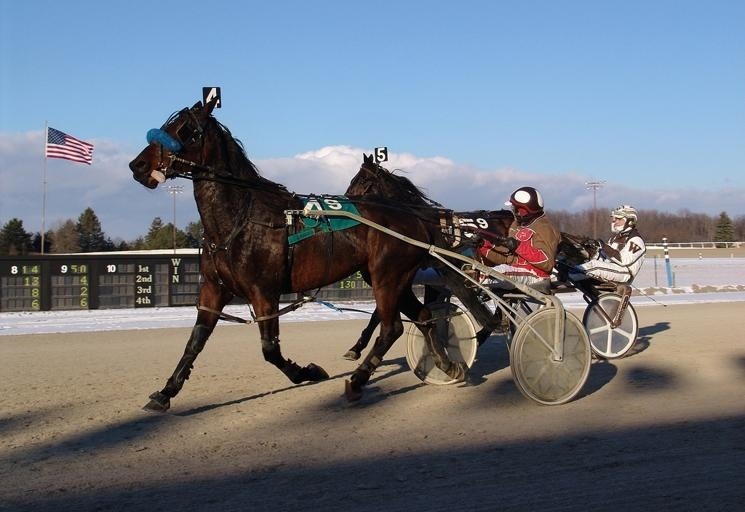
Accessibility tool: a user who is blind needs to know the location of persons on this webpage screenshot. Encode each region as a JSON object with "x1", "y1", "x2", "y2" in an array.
[
  {"x1": 477, "y1": 188, "x2": 562, "y2": 292},
  {"x1": 559, "y1": 207, "x2": 645, "y2": 285}
]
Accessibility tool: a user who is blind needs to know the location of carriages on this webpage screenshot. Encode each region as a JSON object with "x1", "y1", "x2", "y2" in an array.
[{"x1": 129, "y1": 94, "x2": 638, "y2": 414}]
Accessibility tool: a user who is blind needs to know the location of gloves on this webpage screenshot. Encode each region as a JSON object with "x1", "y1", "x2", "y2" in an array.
[
  {"x1": 583, "y1": 239, "x2": 603, "y2": 251},
  {"x1": 470, "y1": 236, "x2": 483, "y2": 248},
  {"x1": 501, "y1": 237, "x2": 520, "y2": 251}
]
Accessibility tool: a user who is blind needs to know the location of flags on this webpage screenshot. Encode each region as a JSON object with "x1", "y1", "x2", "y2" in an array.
[{"x1": 45, "y1": 126, "x2": 95, "y2": 166}]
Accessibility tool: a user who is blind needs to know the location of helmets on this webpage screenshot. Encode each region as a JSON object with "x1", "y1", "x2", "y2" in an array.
[
  {"x1": 609, "y1": 205, "x2": 639, "y2": 235},
  {"x1": 504, "y1": 187, "x2": 544, "y2": 226}
]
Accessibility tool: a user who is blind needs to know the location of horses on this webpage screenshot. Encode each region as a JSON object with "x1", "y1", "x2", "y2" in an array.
[
  {"x1": 340, "y1": 152, "x2": 518, "y2": 361},
  {"x1": 129, "y1": 96, "x2": 468, "y2": 413}
]
